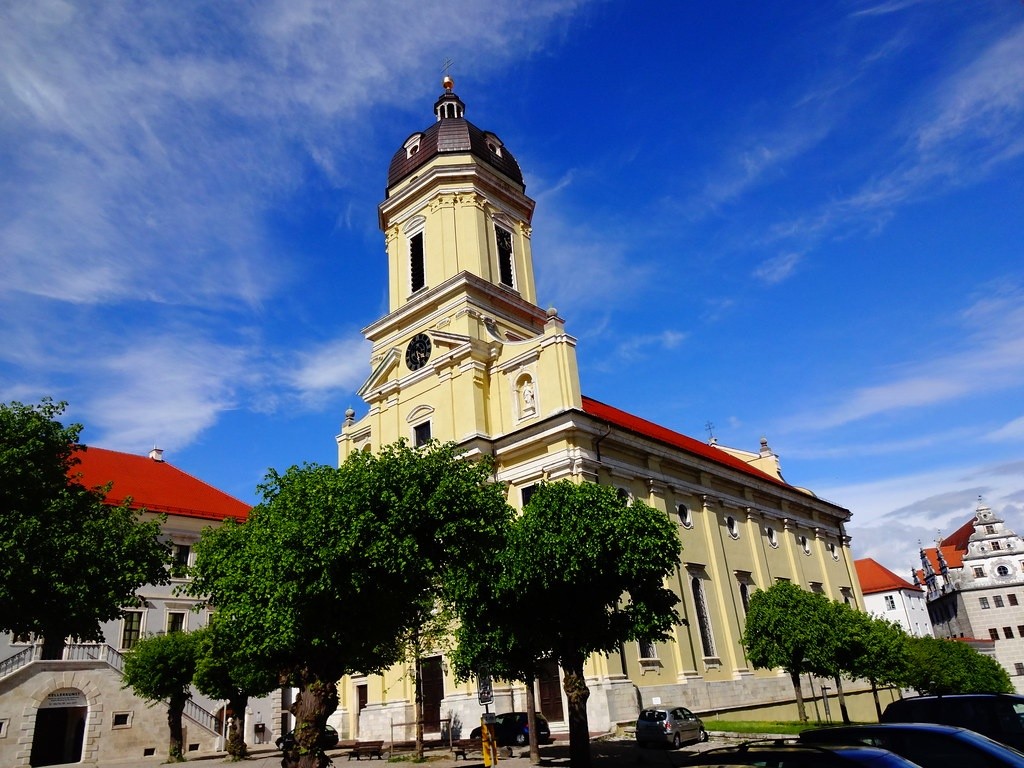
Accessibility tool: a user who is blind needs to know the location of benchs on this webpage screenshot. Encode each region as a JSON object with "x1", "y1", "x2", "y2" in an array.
[
  {"x1": 451, "y1": 737, "x2": 482, "y2": 760},
  {"x1": 348, "y1": 740, "x2": 384, "y2": 761}
]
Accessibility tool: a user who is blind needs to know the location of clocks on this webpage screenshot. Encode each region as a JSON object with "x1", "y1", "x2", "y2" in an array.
[{"x1": 405, "y1": 334, "x2": 432, "y2": 371}]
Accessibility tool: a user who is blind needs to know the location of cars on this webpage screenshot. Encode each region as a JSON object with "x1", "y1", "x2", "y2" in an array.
[
  {"x1": 636, "y1": 705, "x2": 706, "y2": 750},
  {"x1": 672, "y1": 722, "x2": 1024, "y2": 768},
  {"x1": 879, "y1": 692, "x2": 1024, "y2": 754},
  {"x1": 470, "y1": 711, "x2": 551, "y2": 746},
  {"x1": 275, "y1": 724, "x2": 338, "y2": 751}
]
